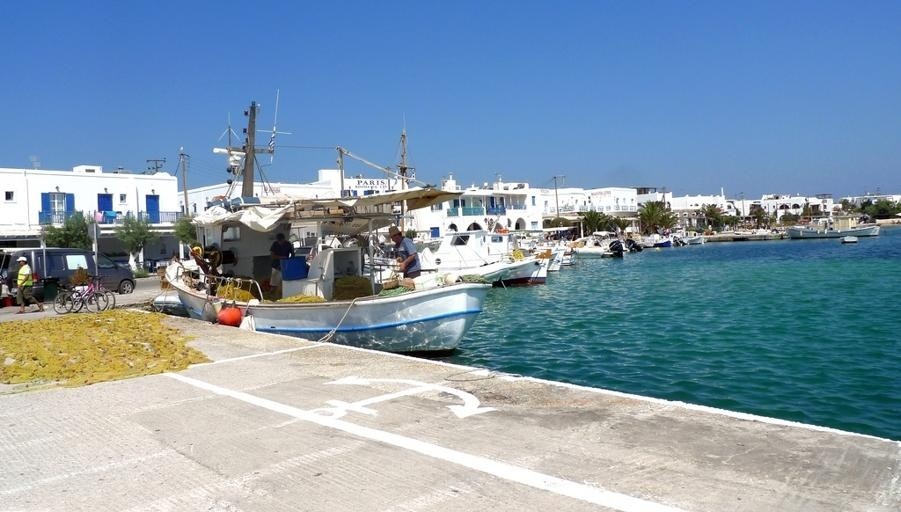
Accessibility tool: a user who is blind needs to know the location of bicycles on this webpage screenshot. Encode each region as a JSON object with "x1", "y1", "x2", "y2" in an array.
[{"x1": 49, "y1": 272, "x2": 120, "y2": 314}]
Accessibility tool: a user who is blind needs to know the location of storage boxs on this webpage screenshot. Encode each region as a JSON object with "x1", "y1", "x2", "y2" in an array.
[{"x1": 278, "y1": 255, "x2": 307, "y2": 281}]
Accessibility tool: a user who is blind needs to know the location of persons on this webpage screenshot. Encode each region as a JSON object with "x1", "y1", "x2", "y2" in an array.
[
  {"x1": 16, "y1": 256, "x2": 43, "y2": 313},
  {"x1": 386, "y1": 226, "x2": 421, "y2": 279},
  {"x1": 269, "y1": 233, "x2": 295, "y2": 298}
]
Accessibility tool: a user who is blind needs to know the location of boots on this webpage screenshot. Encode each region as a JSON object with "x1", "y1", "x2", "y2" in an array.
[
  {"x1": 19, "y1": 305, "x2": 25, "y2": 313},
  {"x1": 38, "y1": 303, "x2": 44, "y2": 312}
]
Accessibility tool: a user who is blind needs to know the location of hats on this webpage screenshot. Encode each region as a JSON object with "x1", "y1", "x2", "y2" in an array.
[
  {"x1": 16, "y1": 255, "x2": 28, "y2": 262},
  {"x1": 387, "y1": 225, "x2": 403, "y2": 239}
]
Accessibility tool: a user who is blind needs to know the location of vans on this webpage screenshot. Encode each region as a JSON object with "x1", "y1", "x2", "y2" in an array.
[{"x1": 0, "y1": 244, "x2": 139, "y2": 305}]
